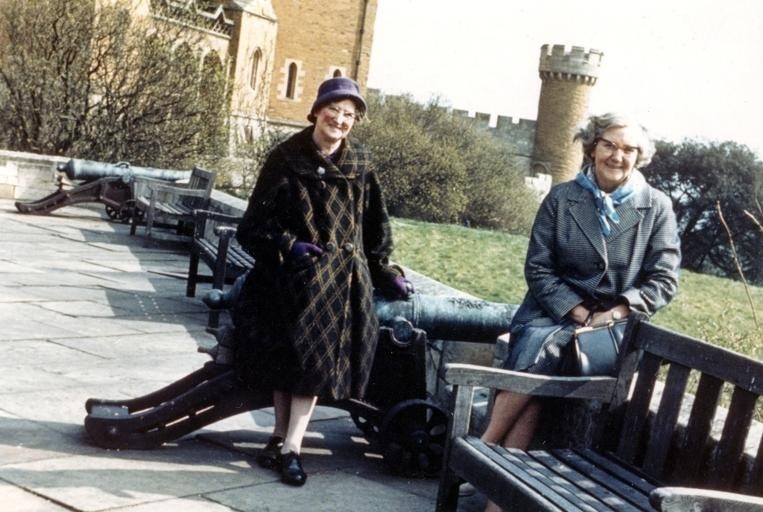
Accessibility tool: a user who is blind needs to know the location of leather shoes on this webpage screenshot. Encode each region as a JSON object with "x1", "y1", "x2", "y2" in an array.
[{"x1": 256, "y1": 436, "x2": 306, "y2": 487}]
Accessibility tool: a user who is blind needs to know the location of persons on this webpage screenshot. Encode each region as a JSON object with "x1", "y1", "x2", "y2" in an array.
[
  {"x1": 236, "y1": 76, "x2": 415, "y2": 486},
  {"x1": 458, "y1": 112, "x2": 683, "y2": 512}
]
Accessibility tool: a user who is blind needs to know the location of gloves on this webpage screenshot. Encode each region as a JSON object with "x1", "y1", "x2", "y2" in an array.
[
  {"x1": 385, "y1": 276, "x2": 415, "y2": 301},
  {"x1": 293, "y1": 242, "x2": 323, "y2": 260}
]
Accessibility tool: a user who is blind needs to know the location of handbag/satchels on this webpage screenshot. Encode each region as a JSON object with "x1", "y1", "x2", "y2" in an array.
[{"x1": 574, "y1": 318, "x2": 634, "y2": 377}]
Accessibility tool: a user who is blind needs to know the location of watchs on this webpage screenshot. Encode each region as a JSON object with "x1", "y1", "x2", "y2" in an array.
[{"x1": 610, "y1": 307, "x2": 622, "y2": 323}]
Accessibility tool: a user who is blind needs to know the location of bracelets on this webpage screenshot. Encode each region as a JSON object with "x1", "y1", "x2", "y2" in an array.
[{"x1": 581, "y1": 310, "x2": 595, "y2": 328}]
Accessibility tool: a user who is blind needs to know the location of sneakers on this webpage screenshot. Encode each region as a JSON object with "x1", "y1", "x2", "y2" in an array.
[{"x1": 458, "y1": 481, "x2": 476, "y2": 496}]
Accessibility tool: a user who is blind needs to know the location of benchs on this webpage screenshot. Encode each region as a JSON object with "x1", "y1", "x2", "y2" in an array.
[
  {"x1": 434, "y1": 309, "x2": 763, "y2": 512},
  {"x1": 129, "y1": 166, "x2": 217, "y2": 248},
  {"x1": 187, "y1": 208, "x2": 257, "y2": 328}
]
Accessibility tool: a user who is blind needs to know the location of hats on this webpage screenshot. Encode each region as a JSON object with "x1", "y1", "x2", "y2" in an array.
[{"x1": 311, "y1": 77, "x2": 367, "y2": 122}]
